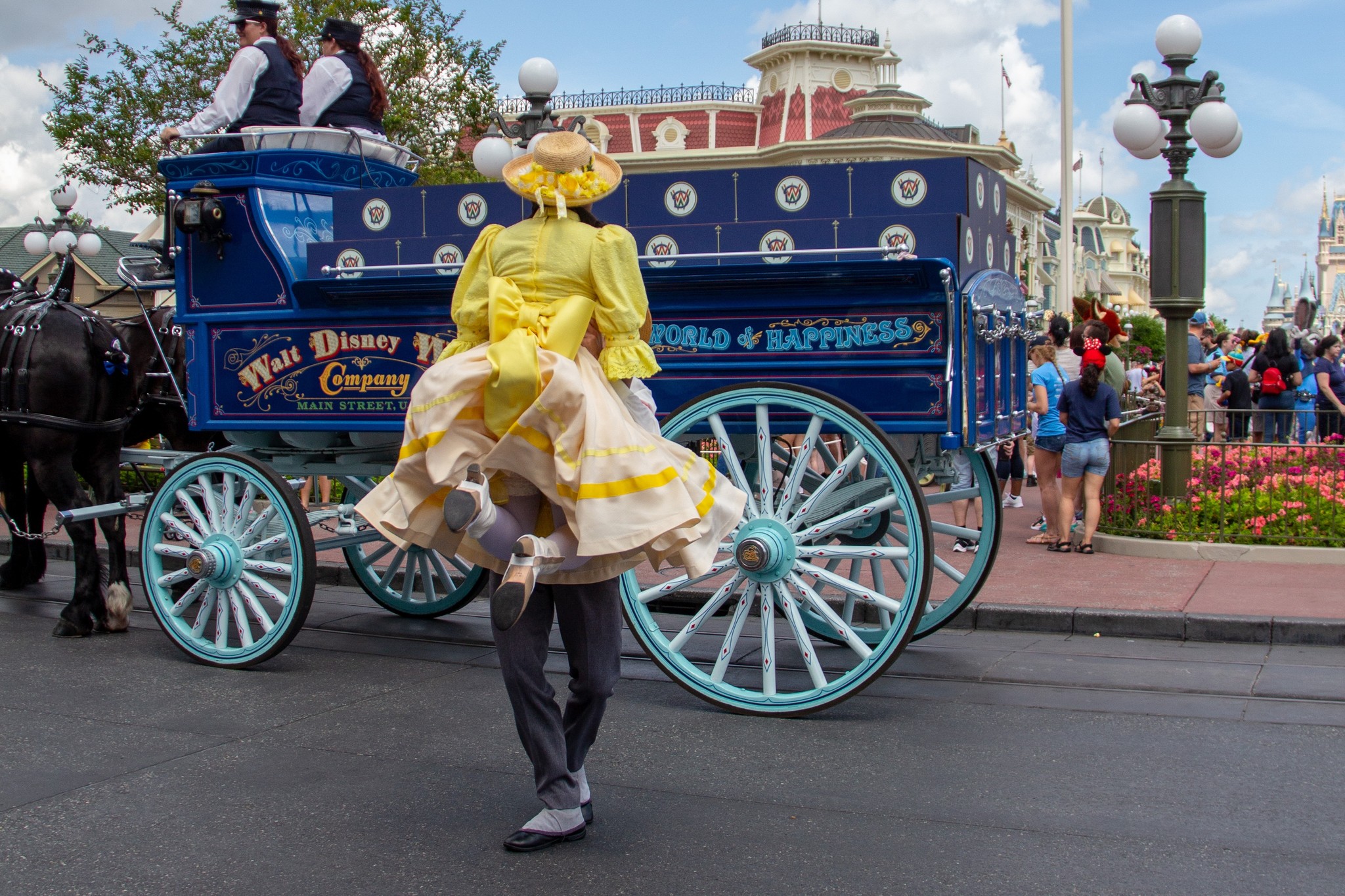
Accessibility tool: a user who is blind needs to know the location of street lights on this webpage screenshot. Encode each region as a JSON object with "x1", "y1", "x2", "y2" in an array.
[
  {"x1": 1124, "y1": 320, "x2": 1133, "y2": 371},
  {"x1": 1113, "y1": 14, "x2": 1244, "y2": 498},
  {"x1": 489, "y1": 56, "x2": 595, "y2": 149},
  {"x1": 24, "y1": 180, "x2": 102, "y2": 303}
]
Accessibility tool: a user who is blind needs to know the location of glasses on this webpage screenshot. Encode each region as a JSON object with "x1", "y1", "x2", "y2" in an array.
[
  {"x1": 1226, "y1": 340, "x2": 1234, "y2": 344},
  {"x1": 1200, "y1": 337, "x2": 1206, "y2": 341},
  {"x1": 1226, "y1": 362, "x2": 1230, "y2": 365},
  {"x1": 1082, "y1": 334, "x2": 1087, "y2": 338},
  {"x1": 237, "y1": 19, "x2": 261, "y2": 31}
]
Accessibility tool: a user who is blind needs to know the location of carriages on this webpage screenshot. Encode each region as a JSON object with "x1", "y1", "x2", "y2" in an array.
[{"x1": 0, "y1": 126, "x2": 1044, "y2": 715}]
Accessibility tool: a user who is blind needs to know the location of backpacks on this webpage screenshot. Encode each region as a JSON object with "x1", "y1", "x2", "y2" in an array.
[{"x1": 1262, "y1": 361, "x2": 1286, "y2": 394}]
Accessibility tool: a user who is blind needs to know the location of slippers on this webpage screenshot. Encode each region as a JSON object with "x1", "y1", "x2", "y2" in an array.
[{"x1": 1026, "y1": 474, "x2": 1037, "y2": 486}]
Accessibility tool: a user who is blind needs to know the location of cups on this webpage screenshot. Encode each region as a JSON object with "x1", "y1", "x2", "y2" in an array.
[{"x1": 1212, "y1": 354, "x2": 1220, "y2": 360}]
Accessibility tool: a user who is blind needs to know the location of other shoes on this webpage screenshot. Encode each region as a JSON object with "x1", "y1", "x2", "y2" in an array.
[
  {"x1": 1031, "y1": 511, "x2": 1045, "y2": 530},
  {"x1": 953, "y1": 539, "x2": 979, "y2": 554}
]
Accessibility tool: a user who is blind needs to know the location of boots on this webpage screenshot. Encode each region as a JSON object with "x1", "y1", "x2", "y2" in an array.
[
  {"x1": 444, "y1": 463, "x2": 497, "y2": 540},
  {"x1": 491, "y1": 534, "x2": 566, "y2": 633}
]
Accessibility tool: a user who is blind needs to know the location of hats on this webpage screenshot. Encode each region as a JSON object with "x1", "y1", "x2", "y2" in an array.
[
  {"x1": 313, "y1": 18, "x2": 362, "y2": 43},
  {"x1": 1221, "y1": 352, "x2": 1243, "y2": 366},
  {"x1": 502, "y1": 131, "x2": 624, "y2": 219},
  {"x1": 1137, "y1": 360, "x2": 1145, "y2": 364},
  {"x1": 1073, "y1": 338, "x2": 1111, "y2": 368},
  {"x1": 1189, "y1": 312, "x2": 1206, "y2": 324},
  {"x1": 1027, "y1": 336, "x2": 1051, "y2": 360},
  {"x1": 229, "y1": 0, "x2": 280, "y2": 24}
]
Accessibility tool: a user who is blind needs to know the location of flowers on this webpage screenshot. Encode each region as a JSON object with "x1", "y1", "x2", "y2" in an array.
[{"x1": 1132, "y1": 346, "x2": 1153, "y2": 364}]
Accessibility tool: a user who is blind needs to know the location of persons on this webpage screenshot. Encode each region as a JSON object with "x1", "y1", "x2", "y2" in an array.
[
  {"x1": 779, "y1": 434, "x2": 868, "y2": 495},
  {"x1": 952, "y1": 441, "x2": 1015, "y2": 553},
  {"x1": 1027, "y1": 336, "x2": 1069, "y2": 543},
  {"x1": 353, "y1": 132, "x2": 750, "y2": 629},
  {"x1": 298, "y1": 18, "x2": 388, "y2": 143},
  {"x1": 485, "y1": 308, "x2": 661, "y2": 850},
  {"x1": 152, "y1": 0, "x2": 308, "y2": 280},
  {"x1": 950, "y1": 311, "x2": 1345, "y2": 532},
  {"x1": 1048, "y1": 338, "x2": 1123, "y2": 554},
  {"x1": 299, "y1": 475, "x2": 332, "y2": 521}
]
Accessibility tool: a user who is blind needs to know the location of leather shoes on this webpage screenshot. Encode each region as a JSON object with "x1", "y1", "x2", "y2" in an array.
[
  {"x1": 581, "y1": 802, "x2": 595, "y2": 823},
  {"x1": 502, "y1": 827, "x2": 588, "y2": 853}
]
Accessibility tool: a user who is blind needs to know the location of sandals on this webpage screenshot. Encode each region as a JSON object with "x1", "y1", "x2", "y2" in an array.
[
  {"x1": 1075, "y1": 541, "x2": 1094, "y2": 554},
  {"x1": 1048, "y1": 538, "x2": 1071, "y2": 553},
  {"x1": 1026, "y1": 533, "x2": 1060, "y2": 544}
]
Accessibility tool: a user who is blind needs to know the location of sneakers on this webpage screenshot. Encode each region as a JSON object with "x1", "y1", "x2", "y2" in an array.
[
  {"x1": 153, "y1": 265, "x2": 175, "y2": 280},
  {"x1": 1002, "y1": 492, "x2": 1023, "y2": 508}
]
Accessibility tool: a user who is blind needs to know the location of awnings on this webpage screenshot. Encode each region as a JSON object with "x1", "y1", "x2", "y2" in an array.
[{"x1": 130, "y1": 217, "x2": 164, "y2": 249}]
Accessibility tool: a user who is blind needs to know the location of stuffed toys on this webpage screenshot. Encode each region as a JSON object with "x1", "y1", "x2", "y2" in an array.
[{"x1": 1073, "y1": 296, "x2": 1130, "y2": 348}]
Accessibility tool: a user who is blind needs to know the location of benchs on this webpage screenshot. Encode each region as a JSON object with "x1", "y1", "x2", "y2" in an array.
[{"x1": 240, "y1": 126, "x2": 412, "y2": 169}]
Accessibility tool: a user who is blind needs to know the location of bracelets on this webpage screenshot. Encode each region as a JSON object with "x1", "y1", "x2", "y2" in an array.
[{"x1": 1207, "y1": 362, "x2": 1212, "y2": 370}]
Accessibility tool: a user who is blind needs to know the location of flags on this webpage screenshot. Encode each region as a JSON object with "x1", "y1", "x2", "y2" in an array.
[
  {"x1": 1099, "y1": 154, "x2": 1104, "y2": 166},
  {"x1": 1001, "y1": 58, "x2": 1011, "y2": 88},
  {"x1": 1072, "y1": 157, "x2": 1082, "y2": 172}
]
